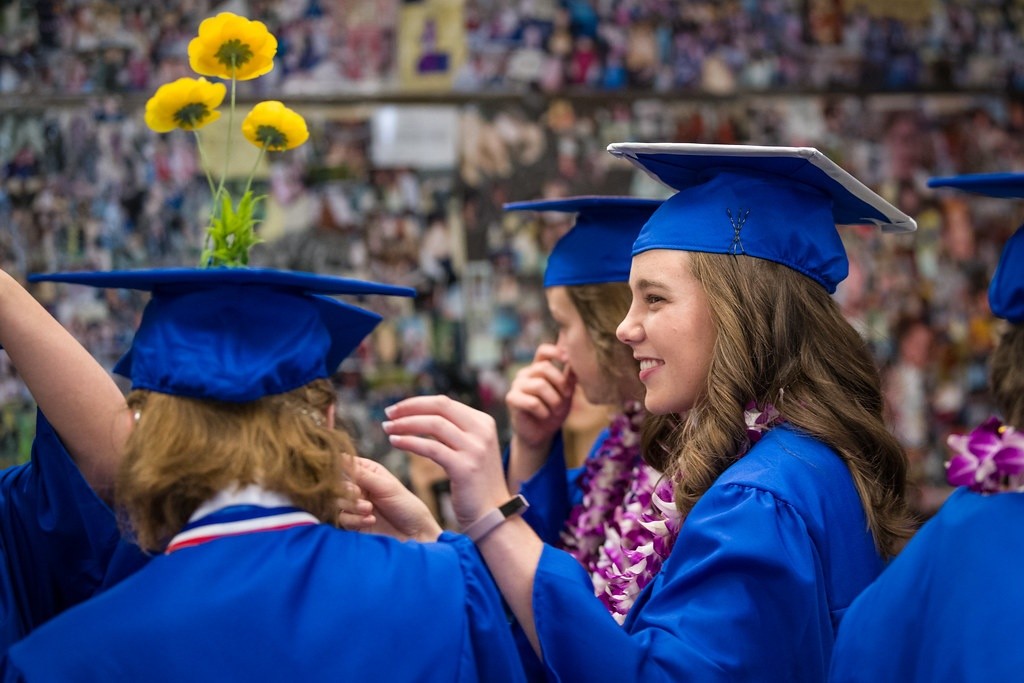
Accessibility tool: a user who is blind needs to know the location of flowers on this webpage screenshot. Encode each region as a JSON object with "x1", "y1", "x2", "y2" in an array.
[{"x1": 145, "y1": 12, "x2": 309, "y2": 268}]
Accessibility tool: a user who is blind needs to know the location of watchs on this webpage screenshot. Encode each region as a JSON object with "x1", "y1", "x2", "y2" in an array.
[{"x1": 456, "y1": 495, "x2": 529, "y2": 543}]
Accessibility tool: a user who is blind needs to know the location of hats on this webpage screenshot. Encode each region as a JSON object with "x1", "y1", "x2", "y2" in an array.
[
  {"x1": 608, "y1": 142, "x2": 917, "y2": 294},
  {"x1": 926, "y1": 172, "x2": 1024, "y2": 321},
  {"x1": 501, "y1": 196, "x2": 667, "y2": 287},
  {"x1": 27, "y1": 265, "x2": 416, "y2": 407}
]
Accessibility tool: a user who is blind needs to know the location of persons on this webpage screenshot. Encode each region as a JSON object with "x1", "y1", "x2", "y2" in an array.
[
  {"x1": 382, "y1": 143, "x2": 918, "y2": 683},
  {"x1": 502, "y1": 197, "x2": 675, "y2": 627},
  {"x1": 0, "y1": 267, "x2": 538, "y2": 682},
  {"x1": 0, "y1": 251, "x2": 140, "y2": 656},
  {"x1": 0, "y1": 0, "x2": 1024, "y2": 576},
  {"x1": 832, "y1": 177, "x2": 1024, "y2": 683}
]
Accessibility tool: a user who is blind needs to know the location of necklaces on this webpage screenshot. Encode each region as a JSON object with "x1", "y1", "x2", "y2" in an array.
[
  {"x1": 944, "y1": 416, "x2": 1024, "y2": 495},
  {"x1": 561, "y1": 391, "x2": 780, "y2": 628}
]
